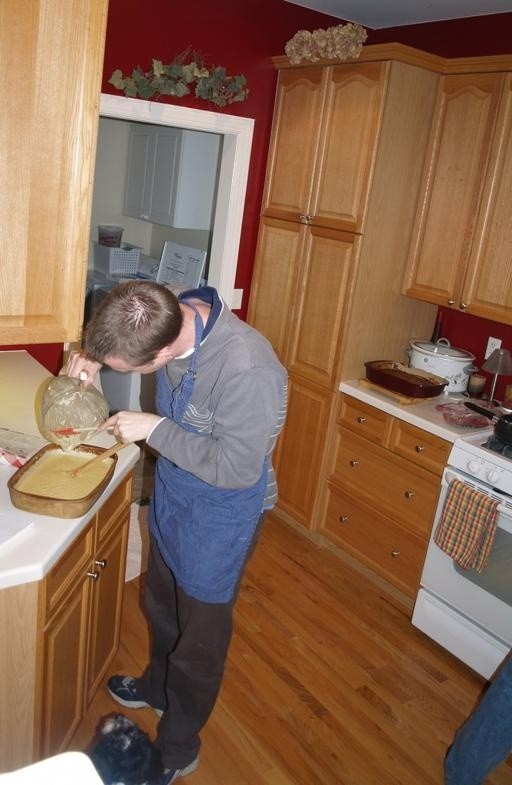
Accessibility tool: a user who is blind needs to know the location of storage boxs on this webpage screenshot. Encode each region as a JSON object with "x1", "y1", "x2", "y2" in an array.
[
  {"x1": 94, "y1": 240, "x2": 140, "y2": 280},
  {"x1": 98, "y1": 225, "x2": 124, "y2": 247}
]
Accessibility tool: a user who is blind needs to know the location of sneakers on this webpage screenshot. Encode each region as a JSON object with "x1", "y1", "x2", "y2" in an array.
[
  {"x1": 163, "y1": 750, "x2": 203, "y2": 785},
  {"x1": 106, "y1": 675, "x2": 164, "y2": 717}
]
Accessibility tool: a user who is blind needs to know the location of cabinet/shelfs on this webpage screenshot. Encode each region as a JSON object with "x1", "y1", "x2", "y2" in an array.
[
  {"x1": 398, "y1": 64, "x2": 512, "y2": 325},
  {"x1": 262, "y1": 63, "x2": 390, "y2": 234},
  {"x1": 268, "y1": 373, "x2": 336, "y2": 532},
  {"x1": 36, "y1": 471, "x2": 133, "y2": 759},
  {"x1": 0, "y1": 1, "x2": 111, "y2": 346},
  {"x1": 318, "y1": 395, "x2": 453, "y2": 601},
  {"x1": 246, "y1": 218, "x2": 362, "y2": 394},
  {"x1": 121, "y1": 124, "x2": 223, "y2": 231}
]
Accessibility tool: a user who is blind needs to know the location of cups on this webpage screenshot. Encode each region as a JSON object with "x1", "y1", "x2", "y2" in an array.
[
  {"x1": 468, "y1": 375, "x2": 486, "y2": 398},
  {"x1": 41, "y1": 372, "x2": 109, "y2": 450}
]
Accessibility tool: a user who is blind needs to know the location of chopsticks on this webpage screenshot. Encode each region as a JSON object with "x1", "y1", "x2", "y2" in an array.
[{"x1": 75, "y1": 441, "x2": 133, "y2": 477}]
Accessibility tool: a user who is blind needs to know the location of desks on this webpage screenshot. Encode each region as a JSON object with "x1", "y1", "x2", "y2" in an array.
[{"x1": 0, "y1": 350, "x2": 141, "y2": 588}]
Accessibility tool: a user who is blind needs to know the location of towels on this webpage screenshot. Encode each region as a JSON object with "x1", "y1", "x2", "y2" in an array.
[{"x1": 432, "y1": 478, "x2": 502, "y2": 576}]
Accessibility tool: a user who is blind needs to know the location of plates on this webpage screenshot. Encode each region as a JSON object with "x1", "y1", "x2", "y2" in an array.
[
  {"x1": 8, "y1": 443, "x2": 118, "y2": 518},
  {"x1": 436, "y1": 400, "x2": 502, "y2": 428},
  {"x1": 364, "y1": 360, "x2": 449, "y2": 398}
]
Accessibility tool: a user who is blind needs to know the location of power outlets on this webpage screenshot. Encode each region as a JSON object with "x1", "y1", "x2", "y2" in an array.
[{"x1": 484, "y1": 336, "x2": 501, "y2": 360}]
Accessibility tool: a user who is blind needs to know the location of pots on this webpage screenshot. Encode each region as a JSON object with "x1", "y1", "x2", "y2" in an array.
[
  {"x1": 465, "y1": 402, "x2": 512, "y2": 447},
  {"x1": 406, "y1": 338, "x2": 479, "y2": 395}
]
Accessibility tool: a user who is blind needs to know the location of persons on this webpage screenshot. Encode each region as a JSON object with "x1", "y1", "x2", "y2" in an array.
[
  {"x1": 441, "y1": 648, "x2": 511, "y2": 785},
  {"x1": 63, "y1": 279, "x2": 291, "y2": 785}
]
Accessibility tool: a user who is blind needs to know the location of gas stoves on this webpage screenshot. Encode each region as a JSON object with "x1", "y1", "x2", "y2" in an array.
[{"x1": 447, "y1": 430, "x2": 512, "y2": 496}]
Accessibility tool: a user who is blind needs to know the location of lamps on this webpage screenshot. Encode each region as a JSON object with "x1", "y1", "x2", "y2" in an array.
[{"x1": 482, "y1": 349, "x2": 512, "y2": 407}]
